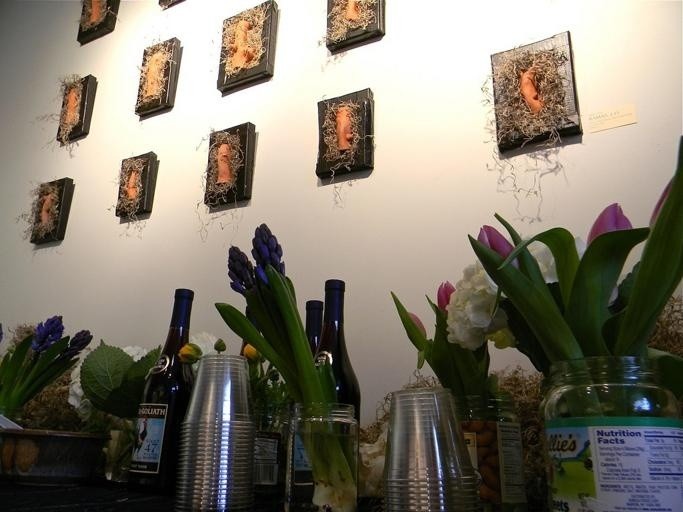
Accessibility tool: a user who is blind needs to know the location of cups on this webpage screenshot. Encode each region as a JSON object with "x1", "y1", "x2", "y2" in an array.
[
  {"x1": 382, "y1": 384, "x2": 479, "y2": 512},
  {"x1": 176, "y1": 354, "x2": 251, "y2": 511}
]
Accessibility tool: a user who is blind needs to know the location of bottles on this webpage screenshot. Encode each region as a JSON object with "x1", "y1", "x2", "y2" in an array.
[
  {"x1": 126, "y1": 288, "x2": 195, "y2": 509},
  {"x1": 306, "y1": 299, "x2": 324, "y2": 363},
  {"x1": 250, "y1": 420, "x2": 289, "y2": 493},
  {"x1": 284, "y1": 280, "x2": 361, "y2": 512}
]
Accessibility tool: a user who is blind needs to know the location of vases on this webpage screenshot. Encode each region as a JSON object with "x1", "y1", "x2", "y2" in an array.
[{"x1": 251, "y1": 414, "x2": 283, "y2": 484}]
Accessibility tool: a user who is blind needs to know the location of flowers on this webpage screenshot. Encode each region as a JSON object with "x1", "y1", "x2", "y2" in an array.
[
  {"x1": 447, "y1": 136, "x2": 683, "y2": 415},
  {"x1": 178, "y1": 338, "x2": 285, "y2": 430},
  {"x1": 0, "y1": 315, "x2": 162, "y2": 452},
  {"x1": 215, "y1": 223, "x2": 355, "y2": 512},
  {"x1": 551, "y1": 442, "x2": 596, "y2": 502},
  {"x1": 390, "y1": 281, "x2": 514, "y2": 421}
]
